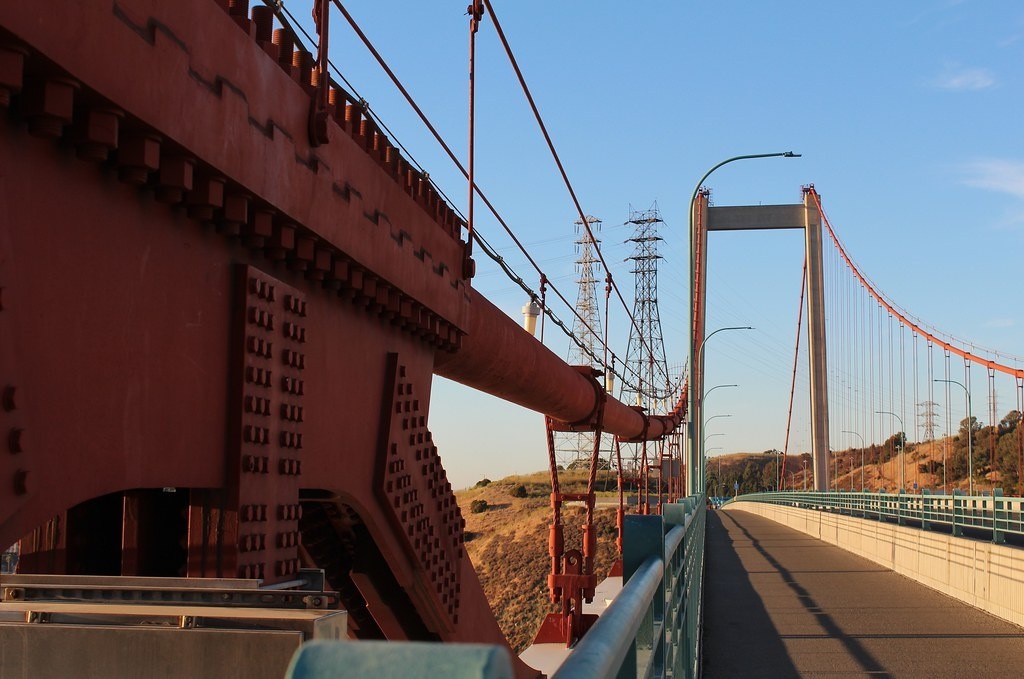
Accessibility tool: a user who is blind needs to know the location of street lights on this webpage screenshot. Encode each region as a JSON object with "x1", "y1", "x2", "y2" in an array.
[
  {"x1": 704, "y1": 434, "x2": 724, "y2": 443},
  {"x1": 876, "y1": 411, "x2": 904, "y2": 488},
  {"x1": 705, "y1": 447, "x2": 723, "y2": 454},
  {"x1": 705, "y1": 385, "x2": 737, "y2": 402},
  {"x1": 687, "y1": 152, "x2": 802, "y2": 495},
  {"x1": 803, "y1": 460, "x2": 807, "y2": 491},
  {"x1": 841, "y1": 431, "x2": 864, "y2": 492},
  {"x1": 934, "y1": 379, "x2": 972, "y2": 496},
  {"x1": 704, "y1": 414, "x2": 732, "y2": 426},
  {"x1": 698, "y1": 326, "x2": 756, "y2": 493}
]
